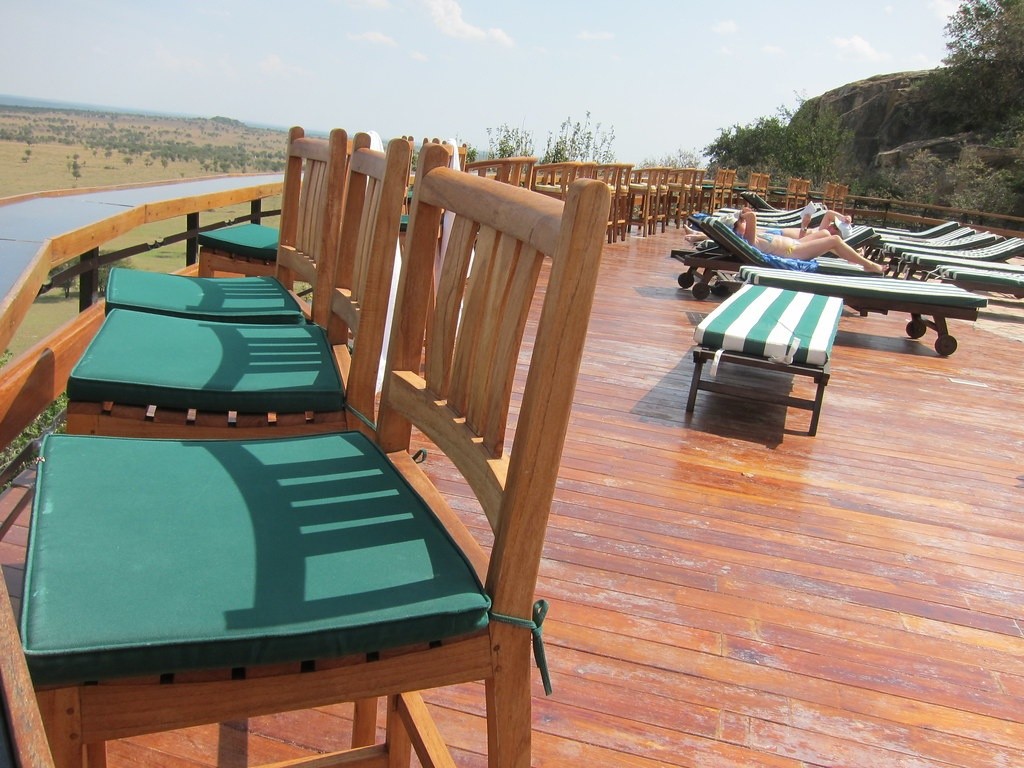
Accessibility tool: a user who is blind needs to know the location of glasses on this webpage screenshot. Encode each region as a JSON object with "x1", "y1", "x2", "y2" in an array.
[{"x1": 834, "y1": 225, "x2": 840, "y2": 233}]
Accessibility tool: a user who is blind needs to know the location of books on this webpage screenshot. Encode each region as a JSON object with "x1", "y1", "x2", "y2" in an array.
[{"x1": 800, "y1": 201, "x2": 816, "y2": 219}]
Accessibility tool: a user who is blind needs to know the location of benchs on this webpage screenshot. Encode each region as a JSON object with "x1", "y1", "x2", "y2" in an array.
[{"x1": 686, "y1": 283, "x2": 844, "y2": 437}]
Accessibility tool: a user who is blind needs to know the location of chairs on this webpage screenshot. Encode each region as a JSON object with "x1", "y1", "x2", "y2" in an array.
[
  {"x1": 21, "y1": 142, "x2": 615, "y2": 768},
  {"x1": 67, "y1": 133, "x2": 411, "y2": 442},
  {"x1": 671, "y1": 191, "x2": 1024, "y2": 355},
  {"x1": 104, "y1": 127, "x2": 347, "y2": 331},
  {"x1": 196, "y1": 134, "x2": 849, "y2": 291}
]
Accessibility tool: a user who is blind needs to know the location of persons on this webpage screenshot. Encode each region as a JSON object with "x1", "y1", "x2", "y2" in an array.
[{"x1": 684, "y1": 208, "x2": 889, "y2": 274}]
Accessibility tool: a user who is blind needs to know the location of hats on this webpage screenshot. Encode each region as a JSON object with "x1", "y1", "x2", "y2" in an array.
[
  {"x1": 834, "y1": 215, "x2": 852, "y2": 239},
  {"x1": 717, "y1": 210, "x2": 741, "y2": 231}
]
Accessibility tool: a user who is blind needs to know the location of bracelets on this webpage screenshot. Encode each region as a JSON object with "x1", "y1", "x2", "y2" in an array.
[{"x1": 802, "y1": 227, "x2": 808, "y2": 232}]
